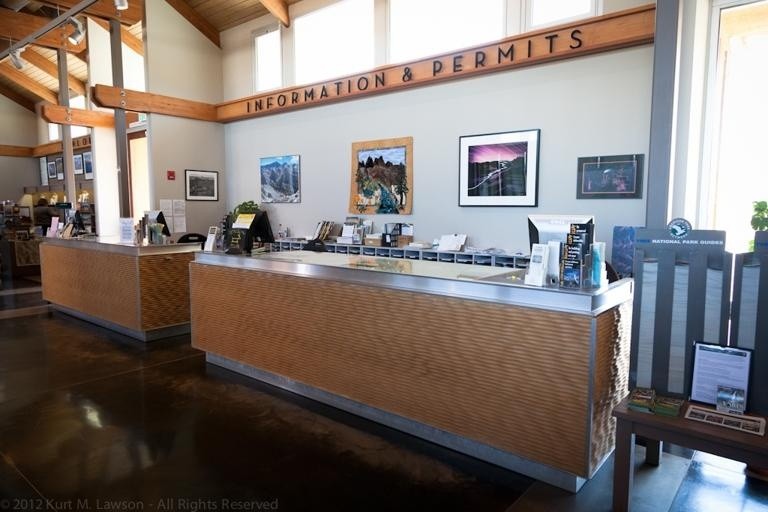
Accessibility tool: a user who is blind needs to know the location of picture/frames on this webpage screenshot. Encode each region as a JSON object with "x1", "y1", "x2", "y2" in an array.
[
  {"x1": 458, "y1": 128, "x2": 540, "y2": 207},
  {"x1": 185, "y1": 170, "x2": 218, "y2": 201},
  {"x1": 39, "y1": 151, "x2": 94, "y2": 186},
  {"x1": 348, "y1": 137, "x2": 413, "y2": 216},
  {"x1": 576, "y1": 153, "x2": 644, "y2": 199}
]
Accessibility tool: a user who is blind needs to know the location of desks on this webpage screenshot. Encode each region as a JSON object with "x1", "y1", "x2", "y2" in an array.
[{"x1": 611, "y1": 392, "x2": 768, "y2": 512}]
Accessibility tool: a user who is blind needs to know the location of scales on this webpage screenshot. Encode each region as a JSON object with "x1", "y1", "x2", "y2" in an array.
[{"x1": 56, "y1": 203, "x2": 71, "y2": 224}]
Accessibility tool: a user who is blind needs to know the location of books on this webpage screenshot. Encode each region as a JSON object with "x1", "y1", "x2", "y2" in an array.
[
  {"x1": 313, "y1": 216, "x2": 372, "y2": 247},
  {"x1": 409, "y1": 239, "x2": 433, "y2": 251},
  {"x1": 205, "y1": 225, "x2": 221, "y2": 253},
  {"x1": 529, "y1": 221, "x2": 596, "y2": 286}
]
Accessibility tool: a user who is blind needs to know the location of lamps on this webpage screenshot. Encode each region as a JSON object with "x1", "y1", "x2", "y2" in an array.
[{"x1": 0, "y1": 0, "x2": 131, "y2": 78}]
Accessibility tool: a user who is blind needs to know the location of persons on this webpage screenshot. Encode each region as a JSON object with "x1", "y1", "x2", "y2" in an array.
[
  {"x1": 33, "y1": 198, "x2": 58, "y2": 237},
  {"x1": 627, "y1": 386, "x2": 687, "y2": 417}
]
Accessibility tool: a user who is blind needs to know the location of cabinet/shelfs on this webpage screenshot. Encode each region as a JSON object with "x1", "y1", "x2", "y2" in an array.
[
  {"x1": 8, "y1": 237, "x2": 44, "y2": 288},
  {"x1": 271, "y1": 238, "x2": 531, "y2": 269}
]
